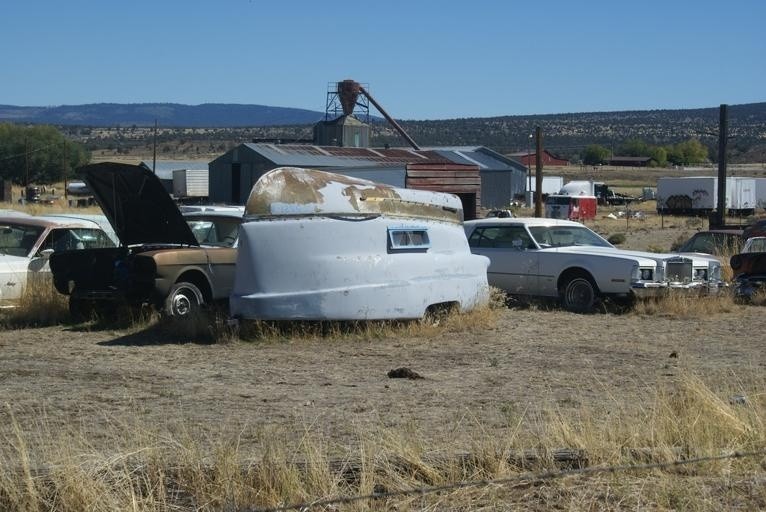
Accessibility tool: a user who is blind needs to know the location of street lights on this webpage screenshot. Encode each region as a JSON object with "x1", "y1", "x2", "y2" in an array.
[{"x1": 526, "y1": 134, "x2": 533, "y2": 191}]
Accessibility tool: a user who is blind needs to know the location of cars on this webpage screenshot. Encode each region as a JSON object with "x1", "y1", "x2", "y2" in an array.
[
  {"x1": 465, "y1": 217, "x2": 722, "y2": 316},
  {"x1": 485, "y1": 209, "x2": 512, "y2": 217},
  {"x1": 0, "y1": 208, "x2": 120, "y2": 313},
  {"x1": 681, "y1": 220, "x2": 765, "y2": 287},
  {"x1": 45, "y1": 161, "x2": 243, "y2": 329}
]
